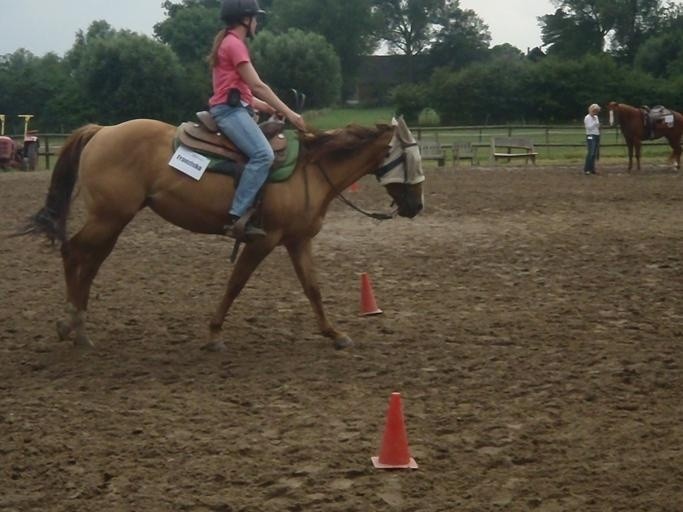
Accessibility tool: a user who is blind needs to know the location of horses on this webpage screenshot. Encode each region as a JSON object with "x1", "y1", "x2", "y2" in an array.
[
  {"x1": 16, "y1": 118, "x2": 424, "y2": 349},
  {"x1": 607, "y1": 101, "x2": 683, "y2": 174}
]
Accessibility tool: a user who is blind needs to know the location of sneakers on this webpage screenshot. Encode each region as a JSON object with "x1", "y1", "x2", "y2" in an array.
[{"x1": 226, "y1": 217, "x2": 268, "y2": 240}]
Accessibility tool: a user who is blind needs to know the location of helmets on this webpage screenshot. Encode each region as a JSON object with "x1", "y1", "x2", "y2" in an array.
[{"x1": 220, "y1": 0, "x2": 266, "y2": 20}]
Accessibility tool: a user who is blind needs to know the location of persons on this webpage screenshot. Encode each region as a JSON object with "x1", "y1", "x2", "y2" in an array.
[
  {"x1": 209, "y1": 0, "x2": 309, "y2": 241},
  {"x1": 584, "y1": 103, "x2": 601, "y2": 175}
]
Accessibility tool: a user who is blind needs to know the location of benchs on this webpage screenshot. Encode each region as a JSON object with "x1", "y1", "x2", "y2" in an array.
[
  {"x1": 491, "y1": 136, "x2": 540, "y2": 167},
  {"x1": 416, "y1": 141, "x2": 446, "y2": 166}
]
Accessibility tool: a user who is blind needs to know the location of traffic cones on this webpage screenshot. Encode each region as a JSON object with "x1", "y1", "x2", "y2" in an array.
[
  {"x1": 348, "y1": 179, "x2": 358, "y2": 190},
  {"x1": 348, "y1": 180, "x2": 360, "y2": 193}
]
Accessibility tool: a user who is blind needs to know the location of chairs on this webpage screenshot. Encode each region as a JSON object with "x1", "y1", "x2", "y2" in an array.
[{"x1": 453, "y1": 140, "x2": 480, "y2": 168}]
